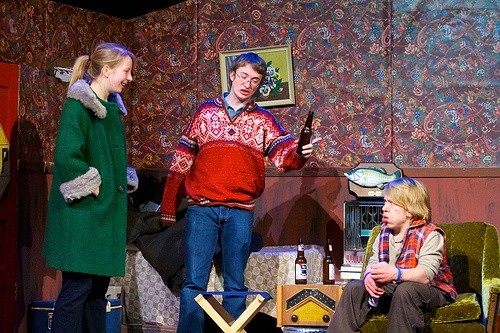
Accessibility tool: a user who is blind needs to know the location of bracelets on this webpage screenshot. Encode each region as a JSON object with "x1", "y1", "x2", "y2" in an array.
[
  {"x1": 395, "y1": 268, "x2": 401, "y2": 282},
  {"x1": 363, "y1": 272, "x2": 371, "y2": 281}
]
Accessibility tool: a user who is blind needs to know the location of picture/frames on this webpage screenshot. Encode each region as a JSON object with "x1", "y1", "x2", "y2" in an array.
[{"x1": 219, "y1": 44, "x2": 295, "y2": 107}]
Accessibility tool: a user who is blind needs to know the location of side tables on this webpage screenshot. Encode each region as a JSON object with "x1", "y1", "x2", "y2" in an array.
[{"x1": 276, "y1": 284, "x2": 345, "y2": 333}]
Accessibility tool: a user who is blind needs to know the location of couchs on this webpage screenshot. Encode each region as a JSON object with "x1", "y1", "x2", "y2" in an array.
[{"x1": 362, "y1": 221, "x2": 500, "y2": 333}]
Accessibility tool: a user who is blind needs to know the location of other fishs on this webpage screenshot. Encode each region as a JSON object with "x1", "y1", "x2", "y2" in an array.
[{"x1": 343, "y1": 165, "x2": 403, "y2": 191}]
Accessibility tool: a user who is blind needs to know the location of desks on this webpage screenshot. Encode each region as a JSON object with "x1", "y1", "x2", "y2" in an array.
[{"x1": 111, "y1": 245, "x2": 324, "y2": 333}]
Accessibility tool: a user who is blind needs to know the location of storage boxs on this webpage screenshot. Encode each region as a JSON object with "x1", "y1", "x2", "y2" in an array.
[{"x1": 29, "y1": 299, "x2": 123, "y2": 333}]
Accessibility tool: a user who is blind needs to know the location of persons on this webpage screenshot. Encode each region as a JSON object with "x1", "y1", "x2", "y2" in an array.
[
  {"x1": 43, "y1": 42, "x2": 139, "y2": 332},
  {"x1": 328, "y1": 178, "x2": 457, "y2": 332},
  {"x1": 161, "y1": 52, "x2": 323, "y2": 332}
]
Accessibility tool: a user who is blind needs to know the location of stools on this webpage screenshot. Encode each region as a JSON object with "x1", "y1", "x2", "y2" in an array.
[{"x1": 190, "y1": 290, "x2": 273, "y2": 333}]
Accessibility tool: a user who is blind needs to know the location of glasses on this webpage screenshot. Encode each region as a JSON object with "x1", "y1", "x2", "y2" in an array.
[{"x1": 234, "y1": 72, "x2": 260, "y2": 88}]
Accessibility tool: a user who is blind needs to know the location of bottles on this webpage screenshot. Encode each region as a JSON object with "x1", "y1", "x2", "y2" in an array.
[
  {"x1": 295, "y1": 238, "x2": 308, "y2": 284},
  {"x1": 323, "y1": 238, "x2": 335, "y2": 284},
  {"x1": 367, "y1": 281, "x2": 388, "y2": 311},
  {"x1": 296, "y1": 110, "x2": 314, "y2": 156}
]
